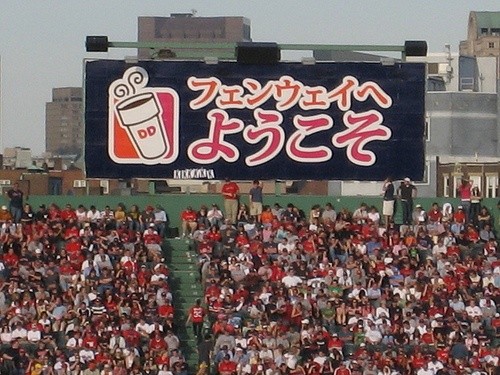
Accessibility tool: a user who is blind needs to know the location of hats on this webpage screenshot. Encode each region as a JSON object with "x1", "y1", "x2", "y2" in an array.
[
  {"x1": 39, "y1": 204, "x2": 45, "y2": 208},
  {"x1": 458, "y1": 206, "x2": 463, "y2": 210},
  {"x1": 404, "y1": 178, "x2": 410, "y2": 182},
  {"x1": 416, "y1": 205, "x2": 421, "y2": 208}
]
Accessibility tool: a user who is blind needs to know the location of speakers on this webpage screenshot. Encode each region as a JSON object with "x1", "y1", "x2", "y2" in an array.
[
  {"x1": 235, "y1": 42, "x2": 279, "y2": 66},
  {"x1": 405, "y1": 40, "x2": 427, "y2": 56},
  {"x1": 86, "y1": 35, "x2": 108, "y2": 52}
]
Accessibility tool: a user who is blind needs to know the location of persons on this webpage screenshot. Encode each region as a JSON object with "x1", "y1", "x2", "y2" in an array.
[
  {"x1": 8, "y1": 183, "x2": 23, "y2": 224},
  {"x1": 397, "y1": 178, "x2": 417, "y2": 225},
  {"x1": 459, "y1": 180, "x2": 472, "y2": 223},
  {"x1": 249, "y1": 180, "x2": 264, "y2": 224},
  {"x1": 0, "y1": 203, "x2": 500, "y2": 375},
  {"x1": 382, "y1": 177, "x2": 395, "y2": 231},
  {"x1": 470, "y1": 185, "x2": 483, "y2": 225},
  {"x1": 222, "y1": 180, "x2": 240, "y2": 225}
]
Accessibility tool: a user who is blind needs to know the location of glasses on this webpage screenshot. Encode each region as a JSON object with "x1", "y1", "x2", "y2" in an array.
[{"x1": 66, "y1": 207, "x2": 71, "y2": 208}]
